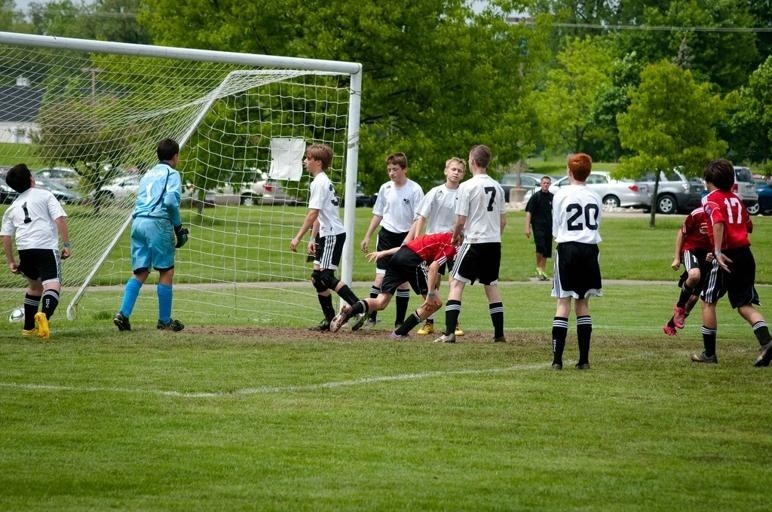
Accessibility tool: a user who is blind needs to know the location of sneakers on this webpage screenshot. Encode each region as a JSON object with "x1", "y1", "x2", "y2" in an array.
[
  {"x1": 34, "y1": 312, "x2": 49, "y2": 338},
  {"x1": 390, "y1": 321, "x2": 409, "y2": 339},
  {"x1": 575, "y1": 363, "x2": 590, "y2": 368},
  {"x1": 662, "y1": 304, "x2": 686, "y2": 336},
  {"x1": 157, "y1": 317, "x2": 184, "y2": 332},
  {"x1": 113, "y1": 311, "x2": 130, "y2": 331},
  {"x1": 691, "y1": 351, "x2": 717, "y2": 364},
  {"x1": 417, "y1": 323, "x2": 433, "y2": 335},
  {"x1": 754, "y1": 339, "x2": 772, "y2": 367},
  {"x1": 552, "y1": 363, "x2": 561, "y2": 369},
  {"x1": 492, "y1": 337, "x2": 506, "y2": 341},
  {"x1": 434, "y1": 321, "x2": 465, "y2": 342},
  {"x1": 310, "y1": 305, "x2": 376, "y2": 333},
  {"x1": 535, "y1": 268, "x2": 550, "y2": 281},
  {"x1": 23, "y1": 328, "x2": 39, "y2": 337}
]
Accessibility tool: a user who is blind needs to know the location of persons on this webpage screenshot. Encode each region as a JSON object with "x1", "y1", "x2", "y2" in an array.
[
  {"x1": 692, "y1": 160, "x2": 771, "y2": 366},
  {"x1": 1, "y1": 163, "x2": 70, "y2": 340},
  {"x1": 526, "y1": 176, "x2": 554, "y2": 280},
  {"x1": 361, "y1": 153, "x2": 424, "y2": 330},
  {"x1": 663, "y1": 207, "x2": 712, "y2": 335},
  {"x1": 432, "y1": 146, "x2": 508, "y2": 341},
  {"x1": 330, "y1": 228, "x2": 464, "y2": 339},
  {"x1": 415, "y1": 158, "x2": 465, "y2": 336},
  {"x1": 548, "y1": 153, "x2": 603, "y2": 368},
  {"x1": 113, "y1": 139, "x2": 189, "y2": 330},
  {"x1": 290, "y1": 143, "x2": 368, "y2": 331}
]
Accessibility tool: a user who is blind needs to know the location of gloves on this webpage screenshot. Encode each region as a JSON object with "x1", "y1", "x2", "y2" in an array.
[{"x1": 174, "y1": 223, "x2": 189, "y2": 248}]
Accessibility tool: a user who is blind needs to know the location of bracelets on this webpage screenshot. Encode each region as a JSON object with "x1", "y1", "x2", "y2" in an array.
[
  {"x1": 309, "y1": 236, "x2": 315, "y2": 240},
  {"x1": 62, "y1": 241, "x2": 68, "y2": 250},
  {"x1": 294, "y1": 237, "x2": 299, "y2": 242},
  {"x1": 428, "y1": 291, "x2": 433, "y2": 296}
]
